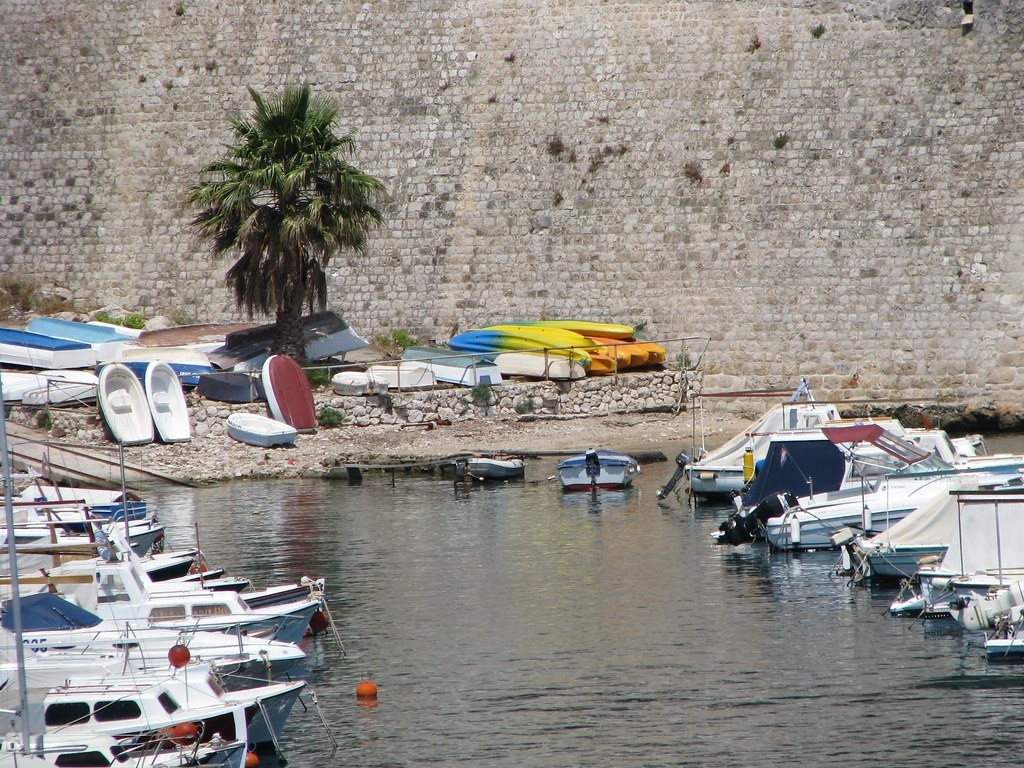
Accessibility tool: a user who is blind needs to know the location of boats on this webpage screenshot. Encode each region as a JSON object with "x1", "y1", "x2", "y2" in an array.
[
  {"x1": 0, "y1": 442, "x2": 349, "y2": 768},
  {"x1": 557, "y1": 446, "x2": 640, "y2": 493},
  {"x1": 666, "y1": 364, "x2": 1023, "y2": 663},
  {"x1": 0, "y1": 297, "x2": 666, "y2": 450},
  {"x1": 463, "y1": 452, "x2": 523, "y2": 479}
]
